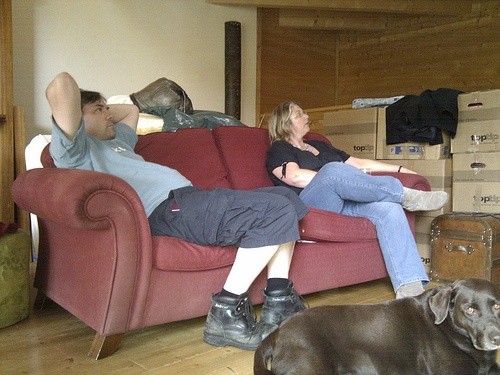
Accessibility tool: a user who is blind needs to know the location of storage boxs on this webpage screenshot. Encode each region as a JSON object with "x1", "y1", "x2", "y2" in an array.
[{"x1": 321, "y1": 90, "x2": 500, "y2": 288}]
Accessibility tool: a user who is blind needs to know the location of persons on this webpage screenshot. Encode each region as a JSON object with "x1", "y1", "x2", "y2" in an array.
[
  {"x1": 46, "y1": 72, "x2": 311, "y2": 351},
  {"x1": 264, "y1": 100, "x2": 449, "y2": 299}
]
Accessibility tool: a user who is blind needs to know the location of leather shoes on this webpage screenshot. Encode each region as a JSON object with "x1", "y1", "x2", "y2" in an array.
[{"x1": 202, "y1": 287, "x2": 310, "y2": 351}]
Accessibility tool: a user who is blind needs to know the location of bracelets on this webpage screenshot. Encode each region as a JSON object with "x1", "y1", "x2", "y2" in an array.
[{"x1": 398, "y1": 166, "x2": 402, "y2": 172}]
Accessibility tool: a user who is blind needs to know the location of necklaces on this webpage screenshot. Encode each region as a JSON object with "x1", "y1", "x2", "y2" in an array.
[{"x1": 305, "y1": 143, "x2": 309, "y2": 151}]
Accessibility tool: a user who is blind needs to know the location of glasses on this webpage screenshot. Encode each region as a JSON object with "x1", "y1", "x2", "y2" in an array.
[{"x1": 290, "y1": 110, "x2": 308, "y2": 120}]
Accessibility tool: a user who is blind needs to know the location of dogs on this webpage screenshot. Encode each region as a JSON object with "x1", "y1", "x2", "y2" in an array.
[{"x1": 254, "y1": 275, "x2": 500, "y2": 375}]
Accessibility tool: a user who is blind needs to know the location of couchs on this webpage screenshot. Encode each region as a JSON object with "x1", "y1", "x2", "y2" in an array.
[{"x1": 10, "y1": 125, "x2": 433, "y2": 362}]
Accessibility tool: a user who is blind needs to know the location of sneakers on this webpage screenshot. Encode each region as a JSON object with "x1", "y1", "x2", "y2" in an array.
[
  {"x1": 396, "y1": 281, "x2": 426, "y2": 300},
  {"x1": 399, "y1": 187, "x2": 448, "y2": 212}
]
